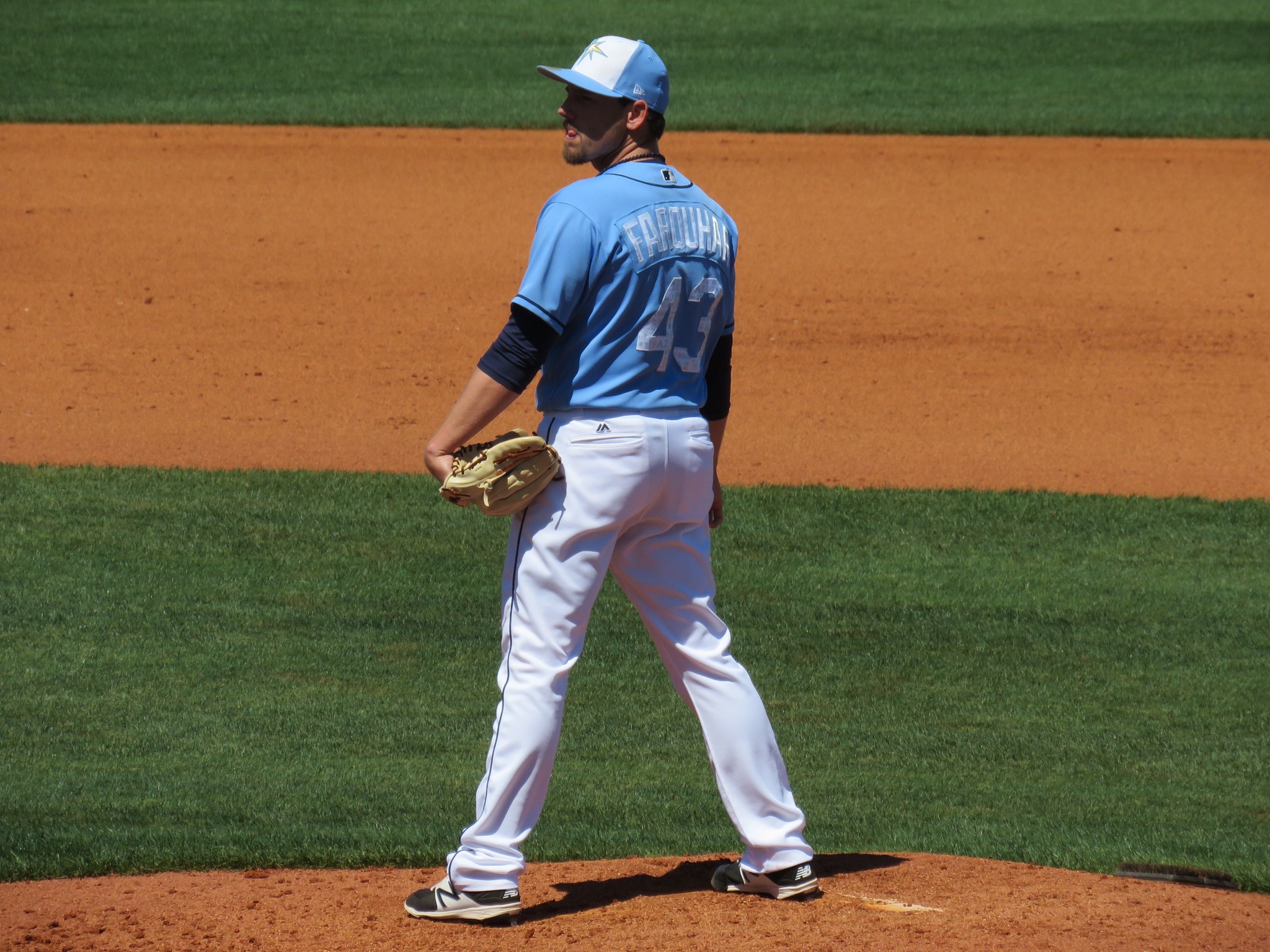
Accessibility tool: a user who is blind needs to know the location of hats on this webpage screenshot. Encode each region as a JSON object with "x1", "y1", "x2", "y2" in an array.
[{"x1": 536, "y1": 34, "x2": 669, "y2": 115}]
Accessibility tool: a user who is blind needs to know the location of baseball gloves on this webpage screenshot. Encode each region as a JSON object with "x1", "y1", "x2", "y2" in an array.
[{"x1": 437, "y1": 429, "x2": 562, "y2": 516}]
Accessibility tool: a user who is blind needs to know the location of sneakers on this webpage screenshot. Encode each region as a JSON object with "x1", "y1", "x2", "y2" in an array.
[
  {"x1": 711, "y1": 858, "x2": 819, "y2": 900},
  {"x1": 404, "y1": 876, "x2": 522, "y2": 920}
]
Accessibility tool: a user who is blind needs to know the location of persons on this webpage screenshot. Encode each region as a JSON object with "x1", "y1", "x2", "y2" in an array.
[{"x1": 366, "y1": 35, "x2": 821, "y2": 924}]
